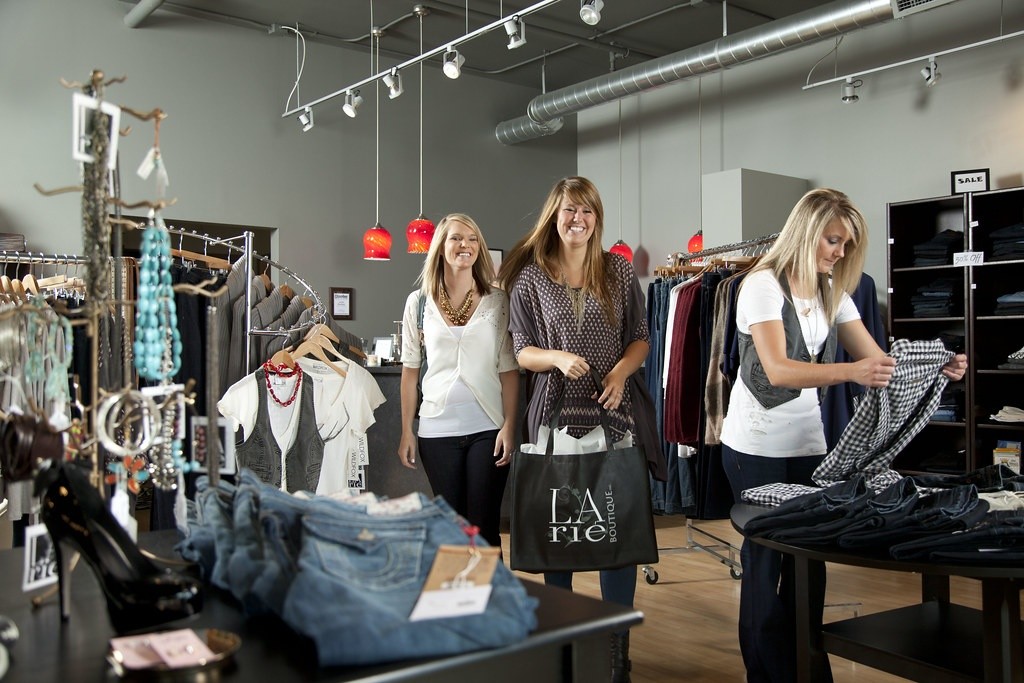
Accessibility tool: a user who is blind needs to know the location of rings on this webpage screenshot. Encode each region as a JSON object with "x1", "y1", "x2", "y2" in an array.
[
  {"x1": 620, "y1": 391, "x2": 623, "y2": 395},
  {"x1": 509, "y1": 452, "x2": 512, "y2": 455}
]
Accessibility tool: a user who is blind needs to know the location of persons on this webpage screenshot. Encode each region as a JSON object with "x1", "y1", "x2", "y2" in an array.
[
  {"x1": 399, "y1": 213, "x2": 524, "y2": 568},
  {"x1": 719, "y1": 189, "x2": 968, "y2": 683},
  {"x1": 498, "y1": 176, "x2": 652, "y2": 683}
]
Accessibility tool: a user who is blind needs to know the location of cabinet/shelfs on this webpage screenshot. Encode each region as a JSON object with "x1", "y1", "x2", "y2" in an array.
[{"x1": 886, "y1": 186, "x2": 1023, "y2": 478}]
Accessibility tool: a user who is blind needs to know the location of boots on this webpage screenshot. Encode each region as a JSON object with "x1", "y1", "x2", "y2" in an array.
[{"x1": 611, "y1": 631, "x2": 631, "y2": 683}]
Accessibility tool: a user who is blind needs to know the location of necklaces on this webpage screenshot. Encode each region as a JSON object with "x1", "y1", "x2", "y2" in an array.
[
  {"x1": 790, "y1": 270, "x2": 818, "y2": 363},
  {"x1": 136, "y1": 222, "x2": 182, "y2": 378},
  {"x1": 81, "y1": 89, "x2": 111, "y2": 300},
  {"x1": 265, "y1": 360, "x2": 302, "y2": 407},
  {"x1": 440, "y1": 281, "x2": 475, "y2": 325},
  {"x1": 557, "y1": 255, "x2": 587, "y2": 335}
]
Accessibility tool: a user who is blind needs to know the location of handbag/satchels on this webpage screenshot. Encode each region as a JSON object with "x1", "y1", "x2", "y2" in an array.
[
  {"x1": 510, "y1": 365, "x2": 659, "y2": 573},
  {"x1": 414, "y1": 288, "x2": 428, "y2": 419}
]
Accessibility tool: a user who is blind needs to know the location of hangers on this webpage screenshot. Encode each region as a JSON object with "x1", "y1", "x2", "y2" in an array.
[
  {"x1": 653, "y1": 230, "x2": 782, "y2": 281},
  {"x1": 0, "y1": 249, "x2": 89, "y2": 308},
  {"x1": 170, "y1": 229, "x2": 368, "y2": 379}
]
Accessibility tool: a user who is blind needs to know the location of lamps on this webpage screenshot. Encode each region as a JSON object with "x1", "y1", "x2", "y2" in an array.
[
  {"x1": 281, "y1": 0, "x2": 605, "y2": 131},
  {"x1": 363, "y1": 27, "x2": 392, "y2": 262},
  {"x1": 802, "y1": 0, "x2": 1024, "y2": 104},
  {"x1": 406, "y1": 5, "x2": 435, "y2": 254},
  {"x1": 687, "y1": 73, "x2": 703, "y2": 263},
  {"x1": 610, "y1": 98, "x2": 634, "y2": 265}
]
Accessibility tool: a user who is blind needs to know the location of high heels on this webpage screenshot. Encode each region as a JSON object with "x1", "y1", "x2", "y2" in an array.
[{"x1": 41, "y1": 463, "x2": 205, "y2": 636}]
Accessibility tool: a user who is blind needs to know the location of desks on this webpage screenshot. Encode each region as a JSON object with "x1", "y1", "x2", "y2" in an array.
[
  {"x1": 730, "y1": 474, "x2": 1024, "y2": 683},
  {"x1": 0, "y1": 530, "x2": 646, "y2": 683}
]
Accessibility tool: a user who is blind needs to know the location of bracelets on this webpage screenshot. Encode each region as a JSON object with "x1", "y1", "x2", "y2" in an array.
[{"x1": 100, "y1": 382, "x2": 183, "y2": 494}]
